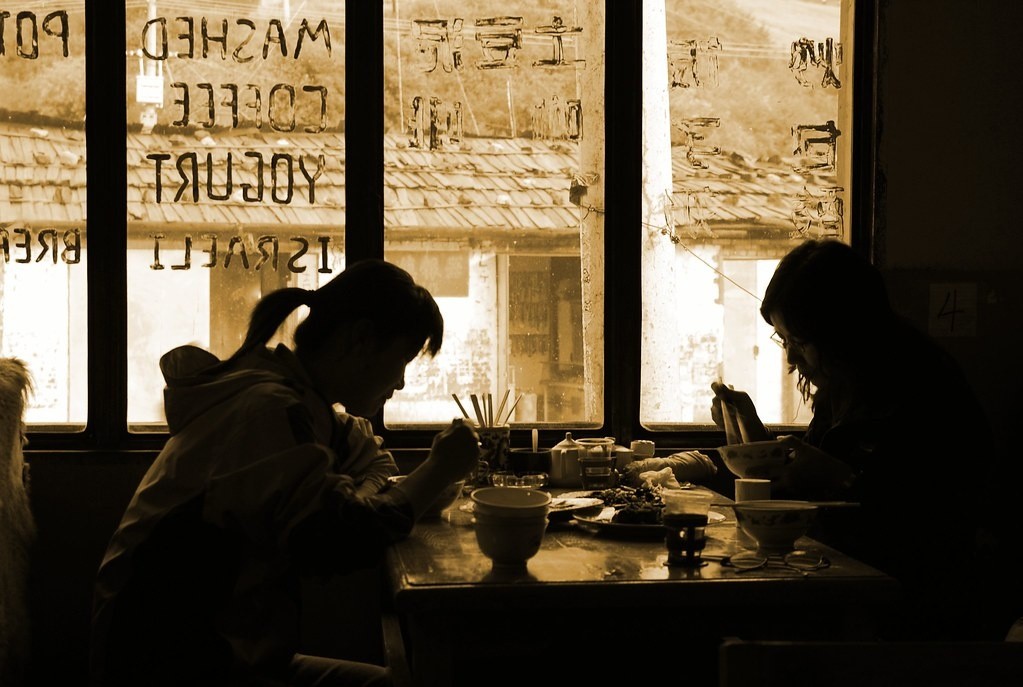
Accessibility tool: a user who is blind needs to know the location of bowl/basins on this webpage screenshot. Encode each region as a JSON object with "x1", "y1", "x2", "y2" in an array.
[
  {"x1": 511, "y1": 448, "x2": 551, "y2": 477},
  {"x1": 717, "y1": 440, "x2": 793, "y2": 479},
  {"x1": 734, "y1": 500, "x2": 817, "y2": 552},
  {"x1": 388, "y1": 476, "x2": 465, "y2": 520},
  {"x1": 589, "y1": 436, "x2": 634, "y2": 474},
  {"x1": 470, "y1": 487, "x2": 553, "y2": 565}
]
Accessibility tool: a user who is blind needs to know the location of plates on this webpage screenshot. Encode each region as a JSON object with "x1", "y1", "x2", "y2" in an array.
[
  {"x1": 547, "y1": 497, "x2": 604, "y2": 513},
  {"x1": 574, "y1": 506, "x2": 726, "y2": 531},
  {"x1": 556, "y1": 490, "x2": 667, "y2": 508}
]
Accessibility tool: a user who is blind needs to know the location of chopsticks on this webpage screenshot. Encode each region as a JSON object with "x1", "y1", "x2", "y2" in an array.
[
  {"x1": 718, "y1": 376, "x2": 749, "y2": 445},
  {"x1": 451, "y1": 389, "x2": 522, "y2": 427},
  {"x1": 710, "y1": 500, "x2": 861, "y2": 508},
  {"x1": 700, "y1": 553, "x2": 829, "y2": 568}
]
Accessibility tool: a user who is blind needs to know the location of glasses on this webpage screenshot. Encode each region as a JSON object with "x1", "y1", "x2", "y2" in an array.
[
  {"x1": 770, "y1": 331, "x2": 813, "y2": 353},
  {"x1": 721, "y1": 547, "x2": 831, "y2": 578}
]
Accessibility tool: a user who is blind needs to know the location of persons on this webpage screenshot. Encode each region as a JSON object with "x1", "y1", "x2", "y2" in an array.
[
  {"x1": 712, "y1": 241, "x2": 992, "y2": 641},
  {"x1": 88, "y1": 262, "x2": 480, "y2": 687}
]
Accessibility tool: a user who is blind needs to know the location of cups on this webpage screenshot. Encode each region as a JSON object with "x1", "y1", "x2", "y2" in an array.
[
  {"x1": 474, "y1": 426, "x2": 510, "y2": 472},
  {"x1": 735, "y1": 479, "x2": 772, "y2": 527},
  {"x1": 662, "y1": 490, "x2": 712, "y2": 551},
  {"x1": 551, "y1": 432, "x2": 586, "y2": 486}
]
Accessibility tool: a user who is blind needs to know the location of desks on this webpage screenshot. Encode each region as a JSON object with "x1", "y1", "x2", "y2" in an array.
[{"x1": 383, "y1": 483, "x2": 892, "y2": 687}]
